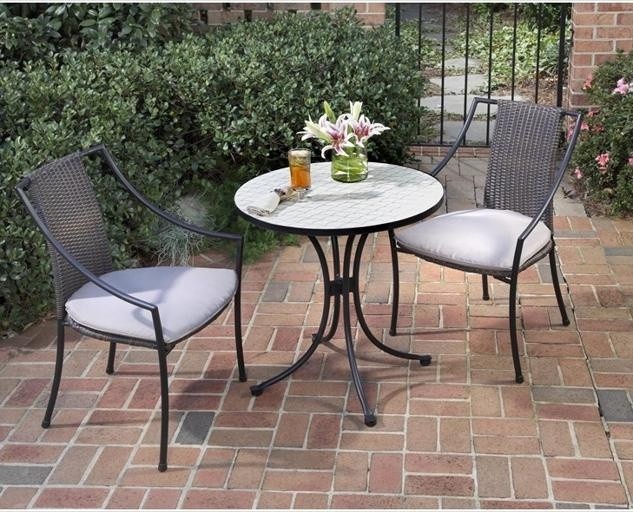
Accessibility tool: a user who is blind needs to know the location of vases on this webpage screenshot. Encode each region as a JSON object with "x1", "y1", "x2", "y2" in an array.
[{"x1": 330, "y1": 143, "x2": 368, "y2": 183}]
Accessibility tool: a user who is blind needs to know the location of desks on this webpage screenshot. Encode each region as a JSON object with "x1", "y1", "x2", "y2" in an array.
[{"x1": 235, "y1": 159, "x2": 445, "y2": 429}]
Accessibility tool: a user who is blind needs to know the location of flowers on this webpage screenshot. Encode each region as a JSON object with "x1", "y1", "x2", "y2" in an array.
[{"x1": 294, "y1": 99, "x2": 391, "y2": 174}]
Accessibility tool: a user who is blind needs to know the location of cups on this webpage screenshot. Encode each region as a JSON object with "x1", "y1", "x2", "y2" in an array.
[{"x1": 287, "y1": 147, "x2": 312, "y2": 192}]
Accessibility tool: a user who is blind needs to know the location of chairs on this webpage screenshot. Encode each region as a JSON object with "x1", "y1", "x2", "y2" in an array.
[
  {"x1": 386, "y1": 93, "x2": 584, "y2": 390},
  {"x1": 14, "y1": 143, "x2": 246, "y2": 473}
]
